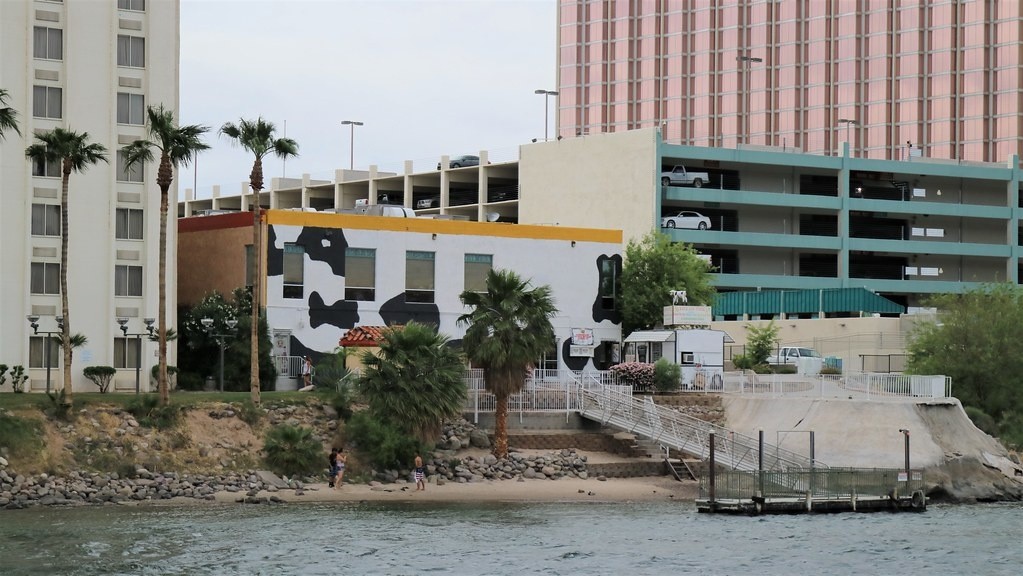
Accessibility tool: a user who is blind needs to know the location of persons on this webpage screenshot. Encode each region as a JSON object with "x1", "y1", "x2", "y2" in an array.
[
  {"x1": 304, "y1": 356, "x2": 313, "y2": 387},
  {"x1": 527, "y1": 362, "x2": 535, "y2": 393},
  {"x1": 329, "y1": 448, "x2": 346, "y2": 489},
  {"x1": 414, "y1": 453, "x2": 425, "y2": 490}
]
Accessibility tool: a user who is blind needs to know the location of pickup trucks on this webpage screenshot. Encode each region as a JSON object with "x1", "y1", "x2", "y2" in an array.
[
  {"x1": 767, "y1": 347, "x2": 825, "y2": 367},
  {"x1": 661, "y1": 165, "x2": 711, "y2": 187}
]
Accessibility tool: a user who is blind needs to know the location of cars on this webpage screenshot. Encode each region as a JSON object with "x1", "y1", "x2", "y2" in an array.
[
  {"x1": 489, "y1": 188, "x2": 516, "y2": 202},
  {"x1": 417, "y1": 192, "x2": 472, "y2": 209},
  {"x1": 355, "y1": 193, "x2": 398, "y2": 207},
  {"x1": 662, "y1": 211, "x2": 712, "y2": 231},
  {"x1": 436, "y1": 155, "x2": 490, "y2": 171}
]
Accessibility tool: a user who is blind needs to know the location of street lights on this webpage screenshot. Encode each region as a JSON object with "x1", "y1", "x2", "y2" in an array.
[
  {"x1": 27, "y1": 313, "x2": 63, "y2": 394},
  {"x1": 906, "y1": 141, "x2": 912, "y2": 157},
  {"x1": 341, "y1": 120, "x2": 364, "y2": 170},
  {"x1": 202, "y1": 313, "x2": 239, "y2": 392},
  {"x1": 837, "y1": 120, "x2": 858, "y2": 143},
  {"x1": 736, "y1": 56, "x2": 762, "y2": 143},
  {"x1": 536, "y1": 90, "x2": 558, "y2": 141},
  {"x1": 118, "y1": 316, "x2": 155, "y2": 395}
]
